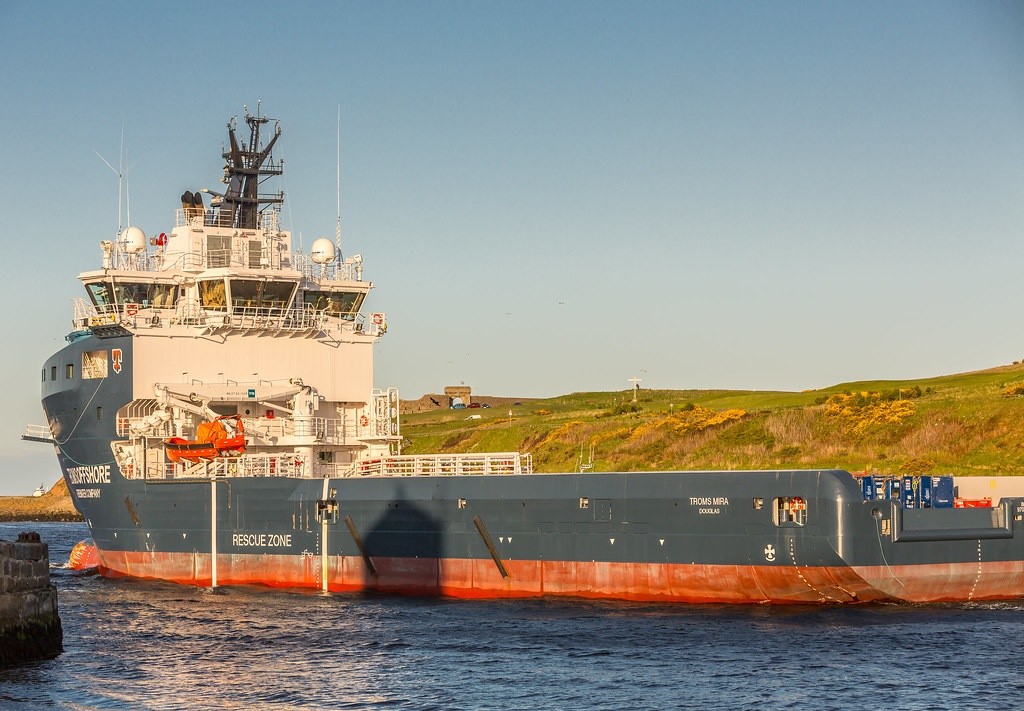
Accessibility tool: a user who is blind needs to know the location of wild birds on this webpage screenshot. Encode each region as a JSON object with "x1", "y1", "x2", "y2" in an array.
[{"x1": 430, "y1": 397, "x2": 441, "y2": 406}]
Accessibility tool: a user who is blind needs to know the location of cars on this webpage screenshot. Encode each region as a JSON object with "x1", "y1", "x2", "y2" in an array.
[
  {"x1": 465, "y1": 402, "x2": 480, "y2": 409},
  {"x1": 481, "y1": 404, "x2": 489, "y2": 408},
  {"x1": 450, "y1": 403, "x2": 465, "y2": 410}
]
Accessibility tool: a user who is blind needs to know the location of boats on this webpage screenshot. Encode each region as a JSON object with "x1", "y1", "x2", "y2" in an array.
[
  {"x1": 19, "y1": 106, "x2": 1024, "y2": 607},
  {"x1": 162, "y1": 415, "x2": 247, "y2": 463}
]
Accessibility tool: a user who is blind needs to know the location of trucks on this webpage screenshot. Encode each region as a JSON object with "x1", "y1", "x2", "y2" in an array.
[{"x1": 466, "y1": 415, "x2": 481, "y2": 420}]
